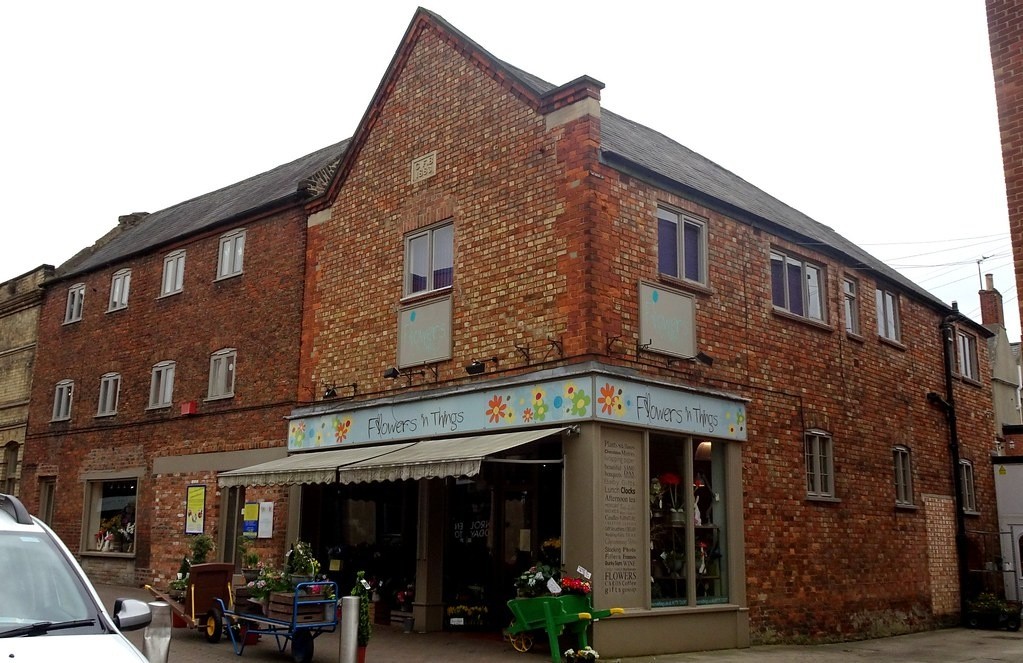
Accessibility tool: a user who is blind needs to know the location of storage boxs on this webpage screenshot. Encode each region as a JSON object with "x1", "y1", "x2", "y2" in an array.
[{"x1": 268, "y1": 591, "x2": 326, "y2": 622}]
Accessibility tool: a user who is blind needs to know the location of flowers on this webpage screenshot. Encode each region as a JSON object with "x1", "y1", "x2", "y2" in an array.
[
  {"x1": 368, "y1": 576, "x2": 388, "y2": 588},
  {"x1": 95, "y1": 515, "x2": 135, "y2": 544},
  {"x1": 287, "y1": 537, "x2": 321, "y2": 576},
  {"x1": 560, "y1": 577, "x2": 592, "y2": 595},
  {"x1": 446, "y1": 605, "x2": 488, "y2": 623},
  {"x1": 396, "y1": 582, "x2": 415, "y2": 611},
  {"x1": 658, "y1": 471, "x2": 701, "y2": 512},
  {"x1": 519, "y1": 563, "x2": 557, "y2": 596},
  {"x1": 246, "y1": 555, "x2": 293, "y2": 600}
]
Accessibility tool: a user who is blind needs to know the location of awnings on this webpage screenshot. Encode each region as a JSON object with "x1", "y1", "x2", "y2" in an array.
[{"x1": 216, "y1": 426, "x2": 572, "y2": 488}]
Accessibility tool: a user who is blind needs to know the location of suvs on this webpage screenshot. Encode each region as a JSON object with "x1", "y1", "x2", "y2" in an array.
[{"x1": 0, "y1": 493, "x2": 152, "y2": 663}]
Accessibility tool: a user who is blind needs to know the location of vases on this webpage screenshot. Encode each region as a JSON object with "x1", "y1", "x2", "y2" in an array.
[
  {"x1": 390, "y1": 610, "x2": 413, "y2": 627},
  {"x1": 670, "y1": 509, "x2": 686, "y2": 527},
  {"x1": 248, "y1": 598, "x2": 268, "y2": 617},
  {"x1": 290, "y1": 574, "x2": 313, "y2": 591},
  {"x1": 370, "y1": 588, "x2": 381, "y2": 602}
]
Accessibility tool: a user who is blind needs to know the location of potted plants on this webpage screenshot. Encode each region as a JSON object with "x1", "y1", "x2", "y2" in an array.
[
  {"x1": 339, "y1": 571, "x2": 370, "y2": 663},
  {"x1": 177, "y1": 532, "x2": 217, "y2": 579},
  {"x1": 168, "y1": 578, "x2": 189, "y2": 604},
  {"x1": 242, "y1": 554, "x2": 263, "y2": 585}
]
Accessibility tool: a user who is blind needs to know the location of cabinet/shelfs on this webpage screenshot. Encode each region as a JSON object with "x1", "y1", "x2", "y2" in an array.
[
  {"x1": 648, "y1": 523, "x2": 720, "y2": 584},
  {"x1": 968, "y1": 527, "x2": 1019, "y2": 622}
]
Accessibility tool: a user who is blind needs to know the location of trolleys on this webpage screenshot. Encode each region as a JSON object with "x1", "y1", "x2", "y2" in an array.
[
  {"x1": 144, "y1": 563, "x2": 236, "y2": 643},
  {"x1": 506, "y1": 594, "x2": 624, "y2": 663},
  {"x1": 211, "y1": 581, "x2": 338, "y2": 663}
]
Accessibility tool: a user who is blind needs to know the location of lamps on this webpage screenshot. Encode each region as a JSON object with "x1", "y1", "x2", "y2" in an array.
[
  {"x1": 670, "y1": 351, "x2": 714, "y2": 369},
  {"x1": 323, "y1": 382, "x2": 357, "y2": 399},
  {"x1": 466, "y1": 357, "x2": 498, "y2": 374},
  {"x1": 384, "y1": 367, "x2": 400, "y2": 381},
  {"x1": 1010, "y1": 439, "x2": 1015, "y2": 449}
]
